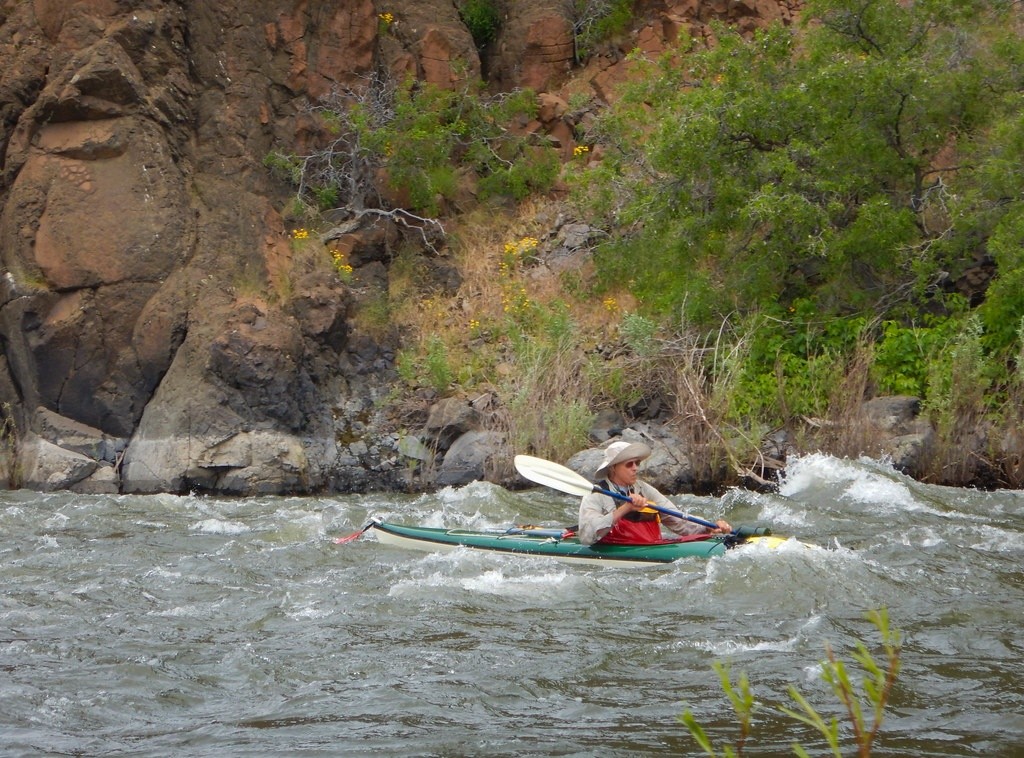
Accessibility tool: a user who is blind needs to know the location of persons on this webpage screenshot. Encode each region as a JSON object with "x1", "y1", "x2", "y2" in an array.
[{"x1": 578, "y1": 442, "x2": 733, "y2": 544}]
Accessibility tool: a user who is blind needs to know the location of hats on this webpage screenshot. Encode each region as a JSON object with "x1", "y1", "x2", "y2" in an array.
[{"x1": 595, "y1": 441, "x2": 652, "y2": 479}]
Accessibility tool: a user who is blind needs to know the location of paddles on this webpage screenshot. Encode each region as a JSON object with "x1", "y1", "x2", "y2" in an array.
[{"x1": 512, "y1": 453, "x2": 787, "y2": 547}]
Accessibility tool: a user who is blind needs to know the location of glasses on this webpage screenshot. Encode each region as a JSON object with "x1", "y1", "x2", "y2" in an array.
[{"x1": 625, "y1": 459, "x2": 641, "y2": 468}]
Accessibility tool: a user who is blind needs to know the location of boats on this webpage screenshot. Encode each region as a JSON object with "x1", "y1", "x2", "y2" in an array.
[{"x1": 369, "y1": 515, "x2": 823, "y2": 567}]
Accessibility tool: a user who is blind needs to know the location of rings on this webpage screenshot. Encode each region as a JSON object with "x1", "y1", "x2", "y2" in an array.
[{"x1": 727, "y1": 525, "x2": 729, "y2": 526}]
